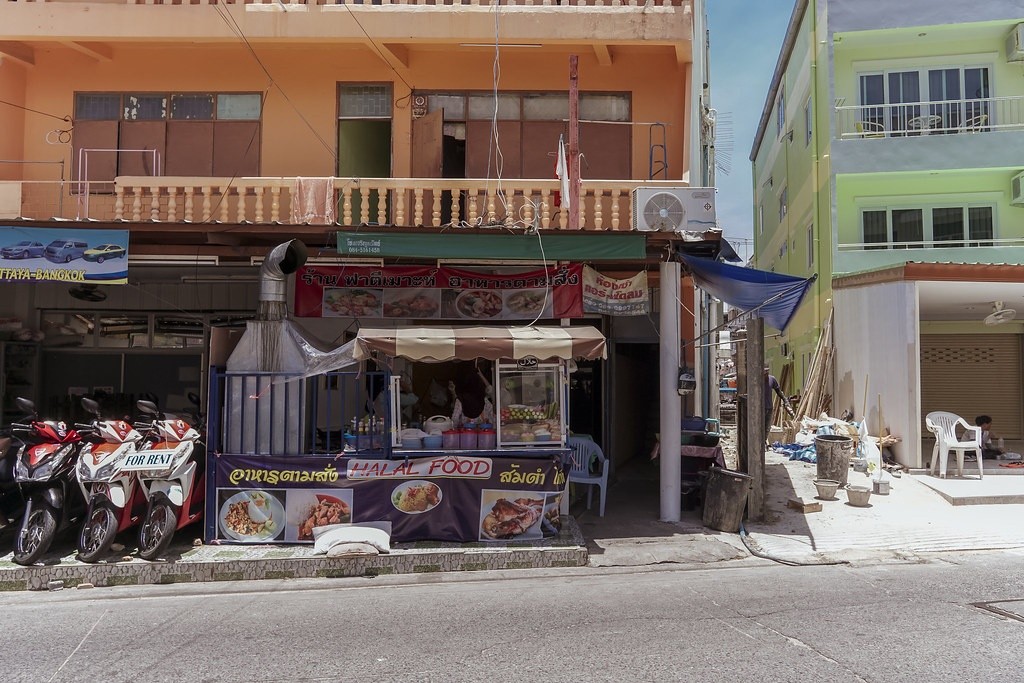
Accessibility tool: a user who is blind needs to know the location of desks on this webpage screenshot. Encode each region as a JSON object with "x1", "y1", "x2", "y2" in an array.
[{"x1": 655, "y1": 442, "x2": 728, "y2": 511}]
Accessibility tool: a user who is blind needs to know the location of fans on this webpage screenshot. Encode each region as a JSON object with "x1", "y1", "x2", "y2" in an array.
[{"x1": 983, "y1": 302, "x2": 1017, "y2": 328}]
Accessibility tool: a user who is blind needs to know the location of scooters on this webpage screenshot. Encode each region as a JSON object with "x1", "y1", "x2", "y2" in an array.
[{"x1": 0, "y1": 397, "x2": 205, "y2": 567}]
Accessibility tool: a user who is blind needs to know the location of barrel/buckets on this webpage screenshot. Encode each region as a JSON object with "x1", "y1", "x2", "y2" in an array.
[
  {"x1": 815, "y1": 435, "x2": 853, "y2": 488},
  {"x1": 846, "y1": 486, "x2": 872, "y2": 506},
  {"x1": 813, "y1": 479, "x2": 842, "y2": 499}
]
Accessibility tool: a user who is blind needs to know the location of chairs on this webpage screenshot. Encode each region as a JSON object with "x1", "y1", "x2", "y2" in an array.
[
  {"x1": 925, "y1": 410, "x2": 984, "y2": 479},
  {"x1": 565, "y1": 437, "x2": 610, "y2": 517}
]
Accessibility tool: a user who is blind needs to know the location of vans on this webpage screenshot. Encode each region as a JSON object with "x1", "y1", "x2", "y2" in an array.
[{"x1": 45, "y1": 240, "x2": 88, "y2": 264}]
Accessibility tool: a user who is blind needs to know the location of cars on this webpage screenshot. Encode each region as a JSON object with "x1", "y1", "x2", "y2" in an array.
[
  {"x1": 1, "y1": 240, "x2": 46, "y2": 260},
  {"x1": 83, "y1": 244, "x2": 126, "y2": 264}
]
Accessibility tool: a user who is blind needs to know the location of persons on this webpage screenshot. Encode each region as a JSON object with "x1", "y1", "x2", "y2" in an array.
[
  {"x1": 373, "y1": 369, "x2": 419, "y2": 420},
  {"x1": 764, "y1": 362, "x2": 792, "y2": 449},
  {"x1": 960, "y1": 416, "x2": 1005, "y2": 460},
  {"x1": 449, "y1": 367, "x2": 515, "y2": 426}
]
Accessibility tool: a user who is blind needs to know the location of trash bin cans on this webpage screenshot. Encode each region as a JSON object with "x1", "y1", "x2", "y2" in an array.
[
  {"x1": 701, "y1": 465, "x2": 754, "y2": 533},
  {"x1": 814, "y1": 434, "x2": 853, "y2": 487}
]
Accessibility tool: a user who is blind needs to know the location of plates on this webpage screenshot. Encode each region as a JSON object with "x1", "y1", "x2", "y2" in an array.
[
  {"x1": 219, "y1": 491, "x2": 285, "y2": 541},
  {"x1": 391, "y1": 480, "x2": 442, "y2": 515}
]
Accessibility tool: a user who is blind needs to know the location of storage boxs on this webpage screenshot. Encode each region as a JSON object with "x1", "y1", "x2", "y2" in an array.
[{"x1": 496, "y1": 358, "x2": 565, "y2": 448}]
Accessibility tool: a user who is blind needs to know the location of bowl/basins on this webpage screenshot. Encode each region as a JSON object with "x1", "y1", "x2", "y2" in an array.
[
  {"x1": 504, "y1": 290, "x2": 550, "y2": 316},
  {"x1": 455, "y1": 289, "x2": 504, "y2": 319}
]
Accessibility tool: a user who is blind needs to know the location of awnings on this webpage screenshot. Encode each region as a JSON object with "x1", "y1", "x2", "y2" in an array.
[
  {"x1": 353, "y1": 326, "x2": 608, "y2": 362},
  {"x1": 674, "y1": 250, "x2": 819, "y2": 337}
]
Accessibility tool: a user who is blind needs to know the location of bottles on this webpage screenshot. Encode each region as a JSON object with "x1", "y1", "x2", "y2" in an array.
[
  {"x1": 344, "y1": 415, "x2": 384, "y2": 450},
  {"x1": 422, "y1": 416, "x2": 426, "y2": 432},
  {"x1": 418, "y1": 415, "x2": 422, "y2": 430},
  {"x1": 401, "y1": 424, "x2": 407, "y2": 430}
]
